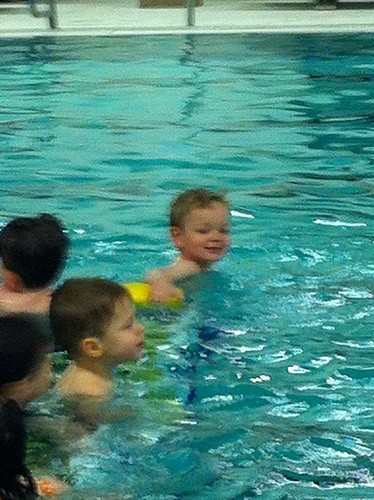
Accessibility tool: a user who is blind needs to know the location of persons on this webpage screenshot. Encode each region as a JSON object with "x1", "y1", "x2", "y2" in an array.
[
  {"x1": 50, "y1": 276, "x2": 147, "y2": 400},
  {"x1": 142, "y1": 189, "x2": 232, "y2": 302},
  {"x1": 0, "y1": 304, "x2": 57, "y2": 499},
  {"x1": 0, "y1": 211, "x2": 72, "y2": 314}
]
